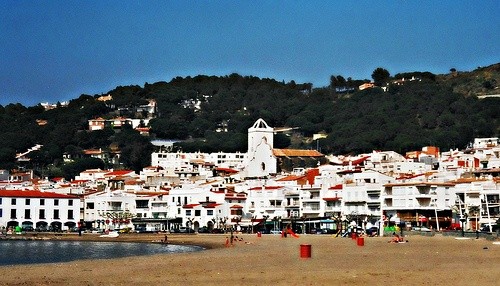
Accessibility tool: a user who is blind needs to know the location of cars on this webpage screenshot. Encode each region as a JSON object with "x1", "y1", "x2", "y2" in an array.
[
  {"x1": 175, "y1": 217, "x2": 499, "y2": 241},
  {"x1": 3, "y1": 223, "x2": 135, "y2": 234}
]
